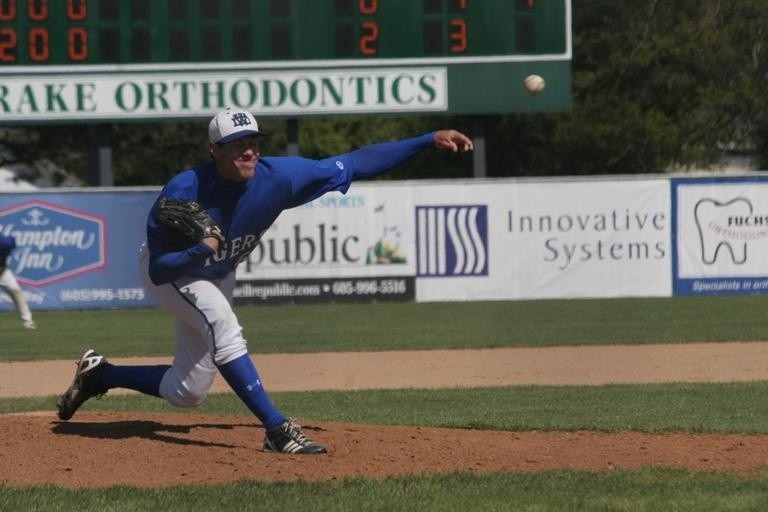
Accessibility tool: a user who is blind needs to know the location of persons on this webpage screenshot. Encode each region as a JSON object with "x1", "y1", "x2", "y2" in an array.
[
  {"x1": 1, "y1": 230, "x2": 40, "y2": 331},
  {"x1": 57, "y1": 105, "x2": 475, "y2": 454}
]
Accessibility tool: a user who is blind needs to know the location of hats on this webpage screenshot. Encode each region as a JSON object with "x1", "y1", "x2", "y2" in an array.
[{"x1": 208, "y1": 106, "x2": 267, "y2": 148}]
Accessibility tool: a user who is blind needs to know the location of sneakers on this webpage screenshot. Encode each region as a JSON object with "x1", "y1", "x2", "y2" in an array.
[
  {"x1": 57, "y1": 345, "x2": 114, "y2": 420},
  {"x1": 262, "y1": 416, "x2": 327, "y2": 454},
  {"x1": 20, "y1": 318, "x2": 37, "y2": 330}
]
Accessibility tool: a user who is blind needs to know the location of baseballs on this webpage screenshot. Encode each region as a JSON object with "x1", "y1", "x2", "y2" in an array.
[{"x1": 526, "y1": 74, "x2": 545, "y2": 94}]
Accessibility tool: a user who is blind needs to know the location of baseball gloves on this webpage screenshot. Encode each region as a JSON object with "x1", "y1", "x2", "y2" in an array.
[{"x1": 150, "y1": 196, "x2": 227, "y2": 251}]
